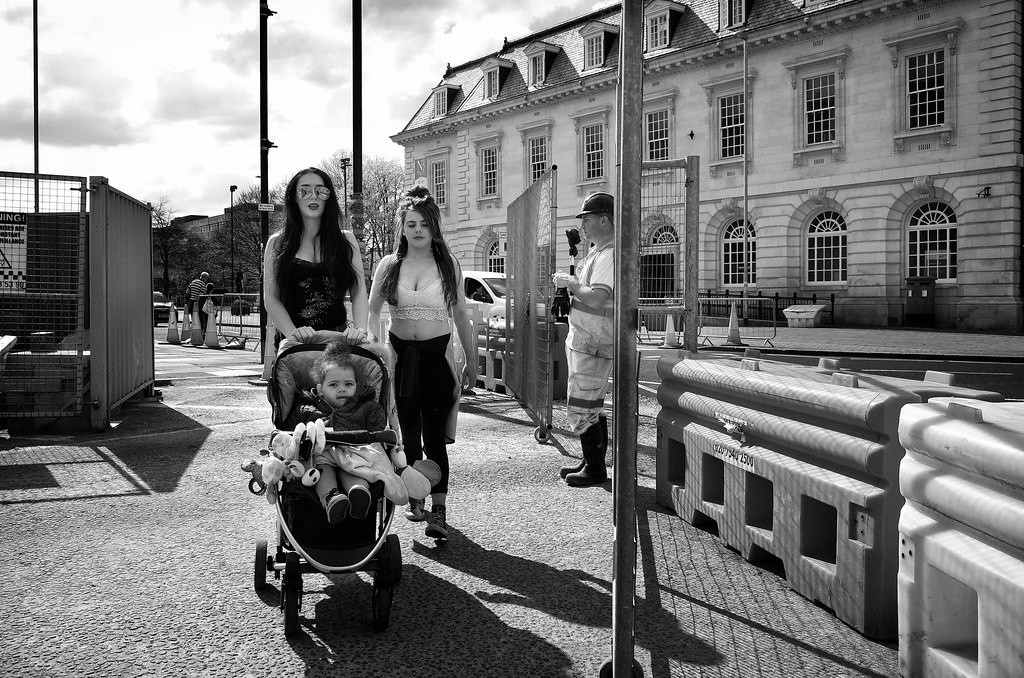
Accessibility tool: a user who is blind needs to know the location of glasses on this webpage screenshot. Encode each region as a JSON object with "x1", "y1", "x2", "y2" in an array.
[{"x1": 296, "y1": 187, "x2": 330, "y2": 201}]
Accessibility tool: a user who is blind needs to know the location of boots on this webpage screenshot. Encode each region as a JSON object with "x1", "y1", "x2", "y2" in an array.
[
  {"x1": 559, "y1": 415, "x2": 608, "y2": 478},
  {"x1": 565, "y1": 422, "x2": 607, "y2": 487}
]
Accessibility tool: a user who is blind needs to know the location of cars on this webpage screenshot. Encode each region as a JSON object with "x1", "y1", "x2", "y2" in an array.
[
  {"x1": 154, "y1": 292, "x2": 178, "y2": 327},
  {"x1": 463, "y1": 271, "x2": 569, "y2": 324}
]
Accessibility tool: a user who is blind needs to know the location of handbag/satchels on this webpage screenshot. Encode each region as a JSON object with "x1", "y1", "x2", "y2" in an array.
[{"x1": 201, "y1": 297, "x2": 217, "y2": 316}]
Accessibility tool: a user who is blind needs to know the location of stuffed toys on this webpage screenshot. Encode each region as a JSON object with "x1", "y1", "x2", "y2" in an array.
[{"x1": 241, "y1": 418, "x2": 326, "y2": 504}]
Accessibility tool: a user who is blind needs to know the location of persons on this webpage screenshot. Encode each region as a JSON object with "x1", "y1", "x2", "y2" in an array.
[
  {"x1": 368, "y1": 184, "x2": 477, "y2": 538},
  {"x1": 552, "y1": 192, "x2": 614, "y2": 488},
  {"x1": 263, "y1": 167, "x2": 370, "y2": 342},
  {"x1": 186, "y1": 272, "x2": 215, "y2": 334},
  {"x1": 284, "y1": 343, "x2": 385, "y2": 524}
]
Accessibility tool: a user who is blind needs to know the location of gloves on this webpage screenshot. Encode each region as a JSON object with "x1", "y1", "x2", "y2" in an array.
[{"x1": 551, "y1": 288, "x2": 570, "y2": 317}]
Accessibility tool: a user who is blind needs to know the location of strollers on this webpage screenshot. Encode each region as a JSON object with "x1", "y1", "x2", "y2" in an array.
[{"x1": 254, "y1": 330, "x2": 403, "y2": 639}]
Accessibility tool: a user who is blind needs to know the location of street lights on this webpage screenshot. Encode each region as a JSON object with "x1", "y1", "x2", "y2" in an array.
[
  {"x1": 230, "y1": 185, "x2": 238, "y2": 293},
  {"x1": 738, "y1": 35, "x2": 748, "y2": 320},
  {"x1": 340, "y1": 158, "x2": 352, "y2": 216}
]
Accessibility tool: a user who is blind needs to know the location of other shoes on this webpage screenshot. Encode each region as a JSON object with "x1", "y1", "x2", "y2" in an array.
[
  {"x1": 348, "y1": 485, "x2": 371, "y2": 520},
  {"x1": 322, "y1": 488, "x2": 349, "y2": 523}
]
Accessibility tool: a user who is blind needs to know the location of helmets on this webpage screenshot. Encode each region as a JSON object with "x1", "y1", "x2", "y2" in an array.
[{"x1": 574, "y1": 192, "x2": 614, "y2": 218}]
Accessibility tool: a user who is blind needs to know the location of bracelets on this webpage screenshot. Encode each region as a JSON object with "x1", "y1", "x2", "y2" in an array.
[{"x1": 355, "y1": 327, "x2": 368, "y2": 336}]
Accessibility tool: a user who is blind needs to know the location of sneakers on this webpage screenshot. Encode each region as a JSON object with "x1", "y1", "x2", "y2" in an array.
[
  {"x1": 425, "y1": 507, "x2": 448, "y2": 538},
  {"x1": 405, "y1": 498, "x2": 425, "y2": 521}
]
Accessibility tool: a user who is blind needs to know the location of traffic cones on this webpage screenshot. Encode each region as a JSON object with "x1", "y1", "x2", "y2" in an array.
[
  {"x1": 720, "y1": 302, "x2": 746, "y2": 345},
  {"x1": 658, "y1": 313, "x2": 682, "y2": 349}
]
